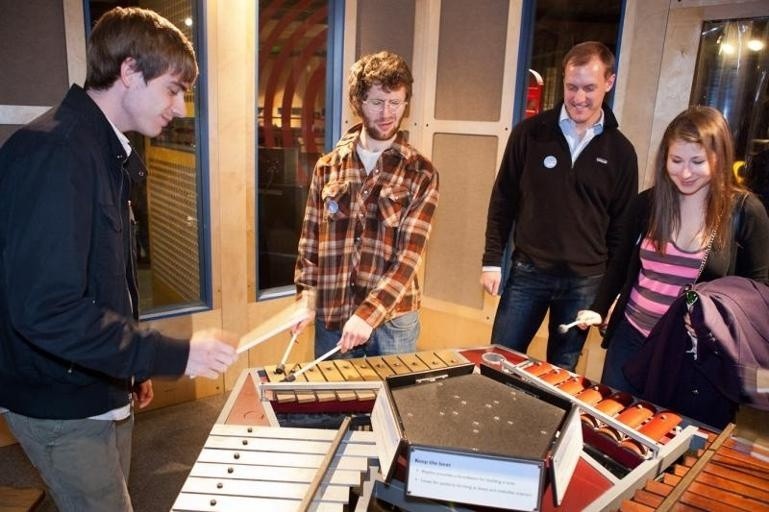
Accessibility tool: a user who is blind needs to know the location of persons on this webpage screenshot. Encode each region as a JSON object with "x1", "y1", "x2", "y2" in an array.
[
  {"x1": 289, "y1": 51, "x2": 440, "y2": 362},
  {"x1": 0, "y1": 6, "x2": 240, "y2": 512}
]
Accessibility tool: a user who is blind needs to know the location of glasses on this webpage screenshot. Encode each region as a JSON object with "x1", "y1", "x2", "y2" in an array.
[{"x1": 362, "y1": 98, "x2": 408, "y2": 110}]
